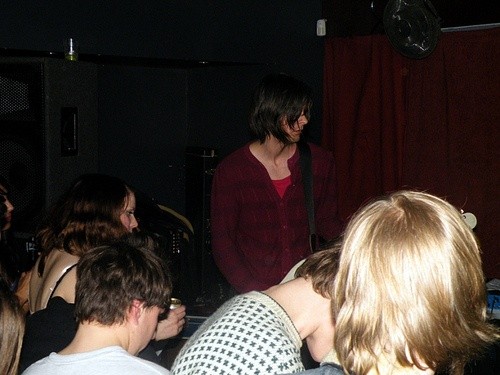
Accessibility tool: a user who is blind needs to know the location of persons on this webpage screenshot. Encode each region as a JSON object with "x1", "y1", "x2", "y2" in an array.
[
  {"x1": 210, "y1": 81, "x2": 348, "y2": 294},
  {"x1": 0, "y1": 173, "x2": 500, "y2": 375}
]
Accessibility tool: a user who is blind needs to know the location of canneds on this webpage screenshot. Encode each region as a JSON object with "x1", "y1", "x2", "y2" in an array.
[{"x1": 167, "y1": 298, "x2": 181, "y2": 310}]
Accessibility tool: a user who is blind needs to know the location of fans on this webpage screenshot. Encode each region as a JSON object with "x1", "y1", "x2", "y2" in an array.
[{"x1": 381, "y1": 0, "x2": 442, "y2": 60}]
